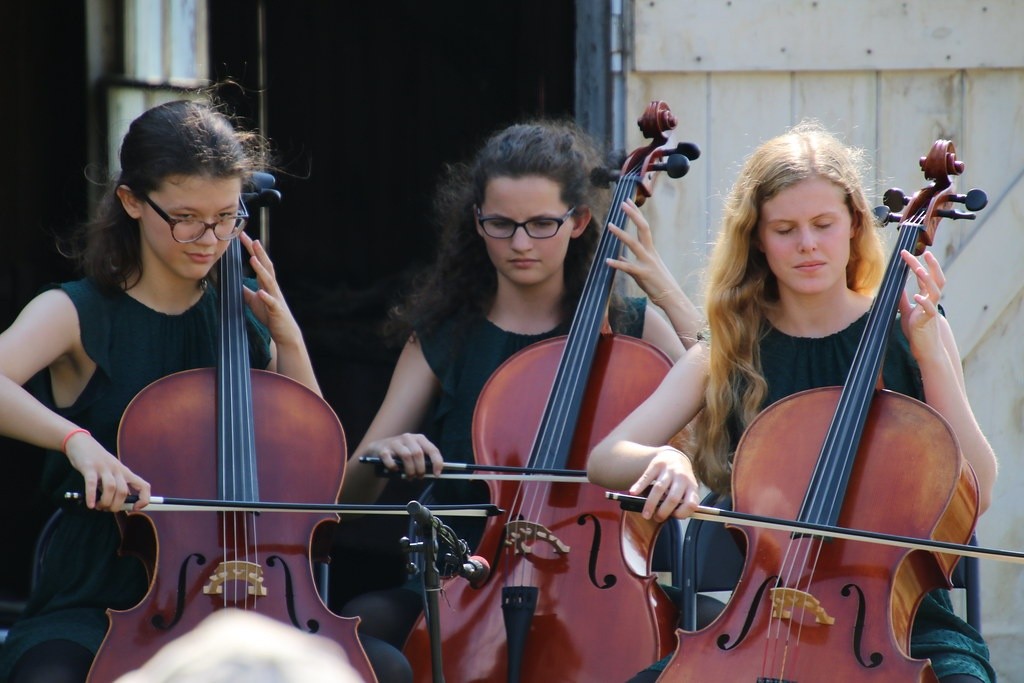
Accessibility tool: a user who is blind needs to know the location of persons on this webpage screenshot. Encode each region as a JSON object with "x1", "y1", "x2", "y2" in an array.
[
  {"x1": 0, "y1": 101, "x2": 415, "y2": 683},
  {"x1": 587, "y1": 118, "x2": 998, "y2": 683},
  {"x1": 336, "y1": 122, "x2": 727, "y2": 652}
]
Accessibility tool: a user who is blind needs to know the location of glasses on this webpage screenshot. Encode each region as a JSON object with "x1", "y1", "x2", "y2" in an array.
[
  {"x1": 132, "y1": 185, "x2": 249, "y2": 243},
  {"x1": 477, "y1": 207, "x2": 575, "y2": 239}
]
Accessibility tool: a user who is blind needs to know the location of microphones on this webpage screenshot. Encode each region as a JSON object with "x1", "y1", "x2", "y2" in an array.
[{"x1": 458, "y1": 555, "x2": 491, "y2": 584}]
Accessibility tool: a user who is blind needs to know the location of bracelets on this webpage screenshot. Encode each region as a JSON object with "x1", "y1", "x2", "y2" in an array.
[{"x1": 63, "y1": 428, "x2": 91, "y2": 454}]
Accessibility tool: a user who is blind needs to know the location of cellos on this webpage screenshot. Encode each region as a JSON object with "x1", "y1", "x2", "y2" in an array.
[
  {"x1": 85, "y1": 169, "x2": 380, "y2": 683},
  {"x1": 654, "y1": 137, "x2": 987, "y2": 683},
  {"x1": 402, "y1": 95, "x2": 703, "y2": 683}
]
